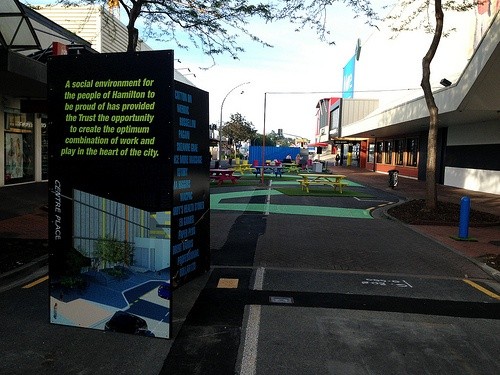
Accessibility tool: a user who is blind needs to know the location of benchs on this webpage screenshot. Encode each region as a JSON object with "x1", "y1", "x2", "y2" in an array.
[
  {"x1": 252, "y1": 171, "x2": 285, "y2": 173},
  {"x1": 296, "y1": 179, "x2": 348, "y2": 185},
  {"x1": 229, "y1": 168, "x2": 256, "y2": 170},
  {"x1": 287, "y1": 168, "x2": 302, "y2": 170},
  {"x1": 210, "y1": 175, "x2": 241, "y2": 178}
]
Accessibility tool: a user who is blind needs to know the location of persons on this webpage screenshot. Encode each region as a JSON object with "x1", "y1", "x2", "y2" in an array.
[
  {"x1": 296, "y1": 153, "x2": 300, "y2": 164},
  {"x1": 7, "y1": 138, "x2": 21, "y2": 174},
  {"x1": 346, "y1": 151, "x2": 352, "y2": 166},
  {"x1": 286, "y1": 153, "x2": 291, "y2": 159},
  {"x1": 335, "y1": 154, "x2": 340, "y2": 167}
]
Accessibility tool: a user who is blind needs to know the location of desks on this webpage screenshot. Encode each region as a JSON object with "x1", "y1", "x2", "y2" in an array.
[
  {"x1": 233, "y1": 164, "x2": 253, "y2": 175},
  {"x1": 282, "y1": 164, "x2": 299, "y2": 173},
  {"x1": 254, "y1": 166, "x2": 289, "y2": 178},
  {"x1": 298, "y1": 174, "x2": 346, "y2": 194},
  {"x1": 210, "y1": 169, "x2": 236, "y2": 185}
]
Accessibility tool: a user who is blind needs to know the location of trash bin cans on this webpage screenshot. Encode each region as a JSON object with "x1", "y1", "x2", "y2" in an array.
[{"x1": 387, "y1": 169, "x2": 401, "y2": 189}]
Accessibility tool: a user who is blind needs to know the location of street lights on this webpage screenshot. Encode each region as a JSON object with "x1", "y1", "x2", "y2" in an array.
[{"x1": 217, "y1": 81, "x2": 252, "y2": 161}]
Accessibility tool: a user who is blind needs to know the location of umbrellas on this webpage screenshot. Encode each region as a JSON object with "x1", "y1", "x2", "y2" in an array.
[{"x1": 306, "y1": 142, "x2": 328, "y2": 162}]
